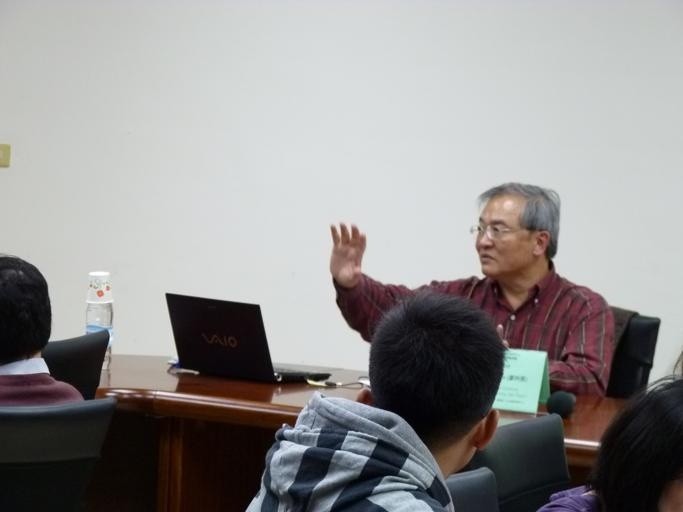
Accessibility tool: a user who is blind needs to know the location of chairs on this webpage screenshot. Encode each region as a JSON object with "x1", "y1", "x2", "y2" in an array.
[
  {"x1": 0, "y1": 397, "x2": 123, "y2": 512},
  {"x1": 438, "y1": 465, "x2": 508, "y2": 512},
  {"x1": 42, "y1": 328, "x2": 110, "y2": 398},
  {"x1": 606, "y1": 307, "x2": 660, "y2": 399},
  {"x1": 477, "y1": 413, "x2": 570, "y2": 512}
]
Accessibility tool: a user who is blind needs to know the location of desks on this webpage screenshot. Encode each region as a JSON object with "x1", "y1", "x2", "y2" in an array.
[{"x1": 89, "y1": 355, "x2": 641, "y2": 512}]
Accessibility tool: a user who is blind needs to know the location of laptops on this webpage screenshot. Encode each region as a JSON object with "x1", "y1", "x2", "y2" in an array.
[{"x1": 165, "y1": 292, "x2": 331, "y2": 385}]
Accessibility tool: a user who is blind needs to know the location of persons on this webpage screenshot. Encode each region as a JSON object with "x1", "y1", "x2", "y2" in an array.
[
  {"x1": 0, "y1": 251, "x2": 86, "y2": 406},
  {"x1": 244, "y1": 291, "x2": 508, "y2": 512},
  {"x1": 533, "y1": 373, "x2": 682, "y2": 512},
  {"x1": 329, "y1": 182, "x2": 615, "y2": 398}
]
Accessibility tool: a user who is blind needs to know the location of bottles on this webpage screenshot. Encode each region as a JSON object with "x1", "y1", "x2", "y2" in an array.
[{"x1": 85, "y1": 270, "x2": 114, "y2": 370}]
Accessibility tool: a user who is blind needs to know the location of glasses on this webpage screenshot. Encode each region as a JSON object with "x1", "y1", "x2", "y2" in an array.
[{"x1": 469, "y1": 223, "x2": 538, "y2": 242}]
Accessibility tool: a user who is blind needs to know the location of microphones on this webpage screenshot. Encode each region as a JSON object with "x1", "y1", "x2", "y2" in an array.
[{"x1": 545, "y1": 390, "x2": 577, "y2": 419}]
